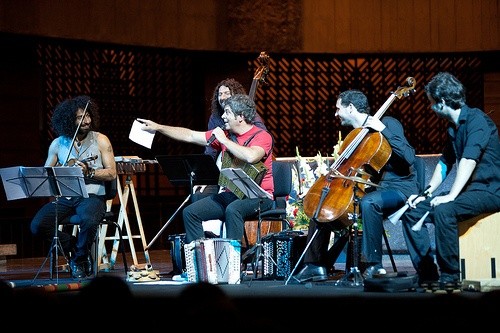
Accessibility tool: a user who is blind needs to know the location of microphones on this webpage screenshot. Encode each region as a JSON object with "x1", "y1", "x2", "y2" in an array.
[{"x1": 206, "y1": 124, "x2": 226, "y2": 147}]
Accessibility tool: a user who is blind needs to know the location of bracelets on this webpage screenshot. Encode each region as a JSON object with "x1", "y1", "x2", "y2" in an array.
[{"x1": 89, "y1": 169, "x2": 95, "y2": 179}]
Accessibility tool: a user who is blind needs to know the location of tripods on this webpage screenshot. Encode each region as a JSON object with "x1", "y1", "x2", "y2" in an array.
[
  {"x1": 230, "y1": 169, "x2": 301, "y2": 288},
  {"x1": 335, "y1": 183, "x2": 364, "y2": 288},
  {"x1": 5, "y1": 167, "x2": 82, "y2": 286}
]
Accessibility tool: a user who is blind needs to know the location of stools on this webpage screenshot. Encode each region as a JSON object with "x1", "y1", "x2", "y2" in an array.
[{"x1": 459, "y1": 211, "x2": 500, "y2": 283}]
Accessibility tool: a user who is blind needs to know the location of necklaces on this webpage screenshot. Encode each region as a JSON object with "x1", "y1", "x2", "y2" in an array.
[{"x1": 75, "y1": 138, "x2": 83, "y2": 146}]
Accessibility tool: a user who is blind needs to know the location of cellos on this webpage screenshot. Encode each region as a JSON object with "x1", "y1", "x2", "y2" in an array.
[
  {"x1": 242, "y1": 51, "x2": 284, "y2": 249},
  {"x1": 302, "y1": 76, "x2": 417, "y2": 232}
]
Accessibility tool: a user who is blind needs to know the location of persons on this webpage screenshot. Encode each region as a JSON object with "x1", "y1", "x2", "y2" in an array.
[
  {"x1": 30, "y1": 96, "x2": 117, "y2": 277},
  {"x1": 286, "y1": 89, "x2": 418, "y2": 286},
  {"x1": 192, "y1": 78, "x2": 268, "y2": 202},
  {"x1": 401, "y1": 72, "x2": 500, "y2": 286},
  {"x1": 137, "y1": 94, "x2": 274, "y2": 282}
]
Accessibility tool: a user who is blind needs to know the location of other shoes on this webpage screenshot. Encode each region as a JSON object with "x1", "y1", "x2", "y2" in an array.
[
  {"x1": 294, "y1": 266, "x2": 328, "y2": 281},
  {"x1": 70, "y1": 261, "x2": 83, "y2": 279},
  {"x1": 438, "y1": 273, "x2": 459, "y2": 289},
  {"x1": 84, "y1": 250, "x2": 93, "y2": 277},
  {"x1": 343, "y1": 264, "x2": 383, "y2": 287},
  {"x1": 409, "y1": 278, "x2": 438, "y2": 291},
  {"x1": 172, "y1": 272, "x2": 187, "y2": 280}
]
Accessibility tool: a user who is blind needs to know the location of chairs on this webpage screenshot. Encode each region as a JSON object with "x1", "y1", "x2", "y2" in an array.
[
  {"x1": 347, "y1": 157, "x2": 425, "y2": 274},
  {"x1": 54, "y1": 172, "x2": 128, "y2": 278},
  {"x1": 239, "y1": 162, "x2": 292, "y2": 279}
]
